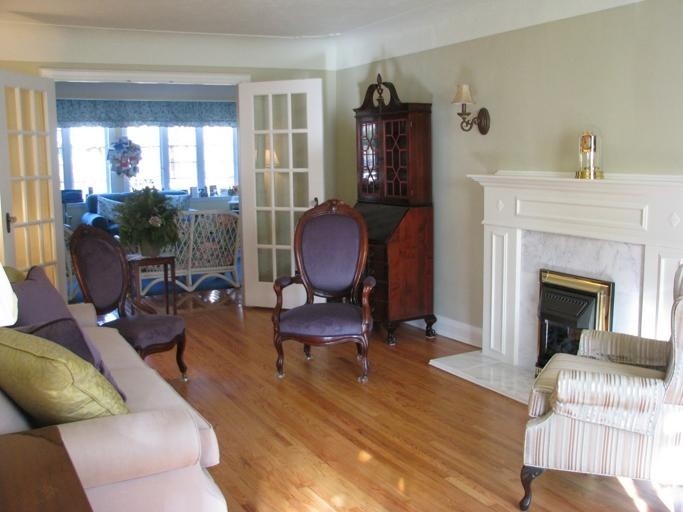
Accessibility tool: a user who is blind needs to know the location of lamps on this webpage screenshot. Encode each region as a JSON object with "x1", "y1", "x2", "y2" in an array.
[{"x1": 449, "y1": 83, "x2": 490, "y2": 137}]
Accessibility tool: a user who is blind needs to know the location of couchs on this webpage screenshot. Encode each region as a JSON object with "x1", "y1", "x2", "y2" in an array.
[
  {"x1": 0, "y1": 301, "x2": 228, "y2": 511},
  {"x1": 80, "y1": 190, "x2": 239, "y2": 296}
]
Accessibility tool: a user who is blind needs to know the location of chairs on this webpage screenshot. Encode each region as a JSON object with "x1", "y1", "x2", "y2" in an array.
[
  {"x1": 516, "y1": 264, "x2": 683, "y2": 511},
  {"x1": 270, "y1": 195, "x2": 378, "y2": 385},
  {"x1": 67, "y1": 224, "x2": 186, "y2": 379}
]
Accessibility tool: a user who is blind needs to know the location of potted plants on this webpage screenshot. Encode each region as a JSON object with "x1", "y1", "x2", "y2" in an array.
[{"x1": 113, "y1": 187, "x2": 180, "y2": 257}]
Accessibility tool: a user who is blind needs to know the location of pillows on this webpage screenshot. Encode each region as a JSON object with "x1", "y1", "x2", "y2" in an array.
[{"x1": 0, "y1": 262, "x2": 128, "y2": 422}]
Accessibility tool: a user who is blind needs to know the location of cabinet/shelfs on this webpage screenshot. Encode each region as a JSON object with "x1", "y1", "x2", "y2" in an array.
[{"x1": 327, "y1": 73, "x2": 436, "y2": 346}]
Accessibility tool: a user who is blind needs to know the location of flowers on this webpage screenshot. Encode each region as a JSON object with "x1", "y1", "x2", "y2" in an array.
[{"x1": 106, "y1": 134, "x2": 141, "y2": 179}]
